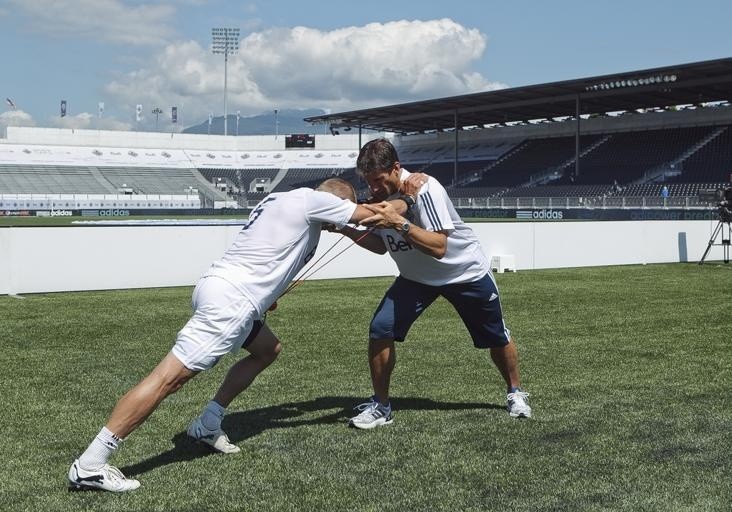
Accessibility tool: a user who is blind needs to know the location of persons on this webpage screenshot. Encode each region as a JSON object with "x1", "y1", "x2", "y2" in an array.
[
  {"x1": 660, "y1": 186, "x2": 670, "y2": 197},
  {"x1": 609, "y1": 180, "x2": 619, "y2": 196},
  {"x1": 62, "y1": 171, "x2": 428, "y2": 494},
  {"x1": 318, "y1": 134, "x2": 535, "y2": 431},
  {"x1": 722, "y1": 172, "x2": 731, "y2": 224}
]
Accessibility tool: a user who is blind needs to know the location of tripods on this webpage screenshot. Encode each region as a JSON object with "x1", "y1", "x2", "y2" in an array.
[{"x1": 698, "y1": 207, "x2": 732, "y2": 265}]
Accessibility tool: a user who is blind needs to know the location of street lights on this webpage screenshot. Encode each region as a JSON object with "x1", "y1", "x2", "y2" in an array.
[
  {"x1": 208, "y1": 24, "x2": 242, "y2": 141},
  {"x1": 150, "y1": 107, "x2": 164, "y2": 132}
]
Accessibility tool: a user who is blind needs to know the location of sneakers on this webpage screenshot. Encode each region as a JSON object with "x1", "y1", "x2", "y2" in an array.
[
  {"x1": 69, "y1": 459, "x2": 140, "y2": 492},
  {"x1": 187, "y1": 419, "x2": 241, "y2": 454},
  {"x1": 506, "y1": 390, "x2": 531, "y2": 419},
  {"x1": 351, "y1": 401, "x2": 394, "y2": 429}
]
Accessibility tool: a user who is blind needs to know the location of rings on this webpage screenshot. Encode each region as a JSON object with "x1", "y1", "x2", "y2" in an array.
[{"x1": 419, "y1": 181, "x2": 425, "y2": 185}]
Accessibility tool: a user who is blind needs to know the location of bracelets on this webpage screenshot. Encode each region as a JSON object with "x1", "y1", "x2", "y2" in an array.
[{"x1": 397, "y1": 192, "x2": 416, "y2": 211}]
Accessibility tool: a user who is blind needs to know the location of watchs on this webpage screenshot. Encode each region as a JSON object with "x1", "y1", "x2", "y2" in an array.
[{"x1": 398, "y1": 219, "x2": 412, "y2": 237}]
